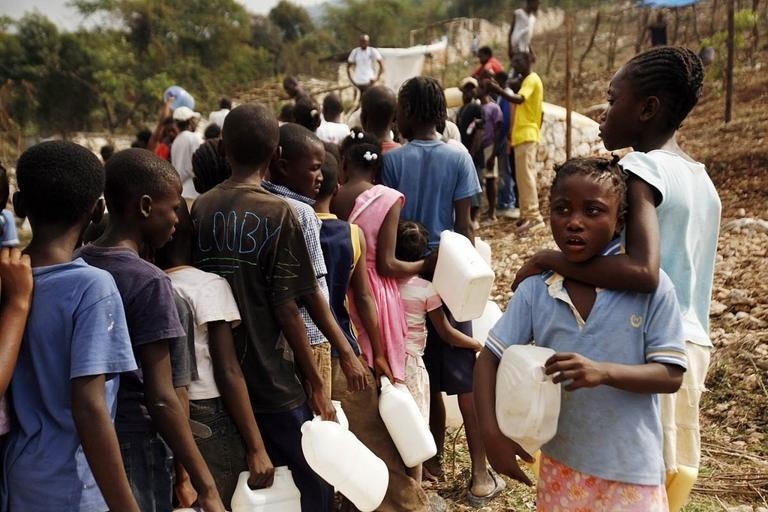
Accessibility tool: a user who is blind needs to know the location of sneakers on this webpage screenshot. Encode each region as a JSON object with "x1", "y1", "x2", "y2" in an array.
[
  {"x1": 514, "y1": 215, "x2": 545, "y2": 235},
  {"x1": 480, "y1": 217, "x2": 498, "y2": 226}
]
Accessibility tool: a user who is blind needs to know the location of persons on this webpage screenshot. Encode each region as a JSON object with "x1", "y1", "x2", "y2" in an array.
[
  {"x1": 471, "y1": 156, "x2": 688, "y2": 510},
  {"x1": 0, "y1": 46, "x2": 546, "y2": 510},
  {"x1": 510, "y1": 46, "x2": 724, "y2": 510},
  {"x1": 507, "y1": 0, "x2": 541, "y2": 77},
  {"x1": 346, "y1": 34, "x2": 387, "y2": 91},
  {"x1": 648, "y1": 14, "x2": 672, "y2": 48}
]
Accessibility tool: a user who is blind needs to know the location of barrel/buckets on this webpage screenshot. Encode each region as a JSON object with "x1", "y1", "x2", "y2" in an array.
[
  {"x1": 472, "y1": 300, "x2": 503, "y2": 359},
  {"x1": 474, "y1": 235, "x2": 492, "y2": 268},
  {"x1": 378, "y1": 375, "x2": 438, "y2": 469},
  {"x1": 230, "y1": 465, "x2": 302, "y2": 512},
  {"x1": 496, "y1": 343, "x2": 562, "y2": 462},
  {"x1": 163, "y1": 85, "x2": 195, "y2": 110},
  {"x1": 432, "y1": 228, "x2": 496, "y2": 324},
  {"x1": 299, "y1": 404, "x2": 390, "y2": 512}
]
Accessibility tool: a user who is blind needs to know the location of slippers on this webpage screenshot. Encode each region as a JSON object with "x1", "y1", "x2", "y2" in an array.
[{"x1": 465, "y1": 468, "x2": 506, "y2": 509}]
